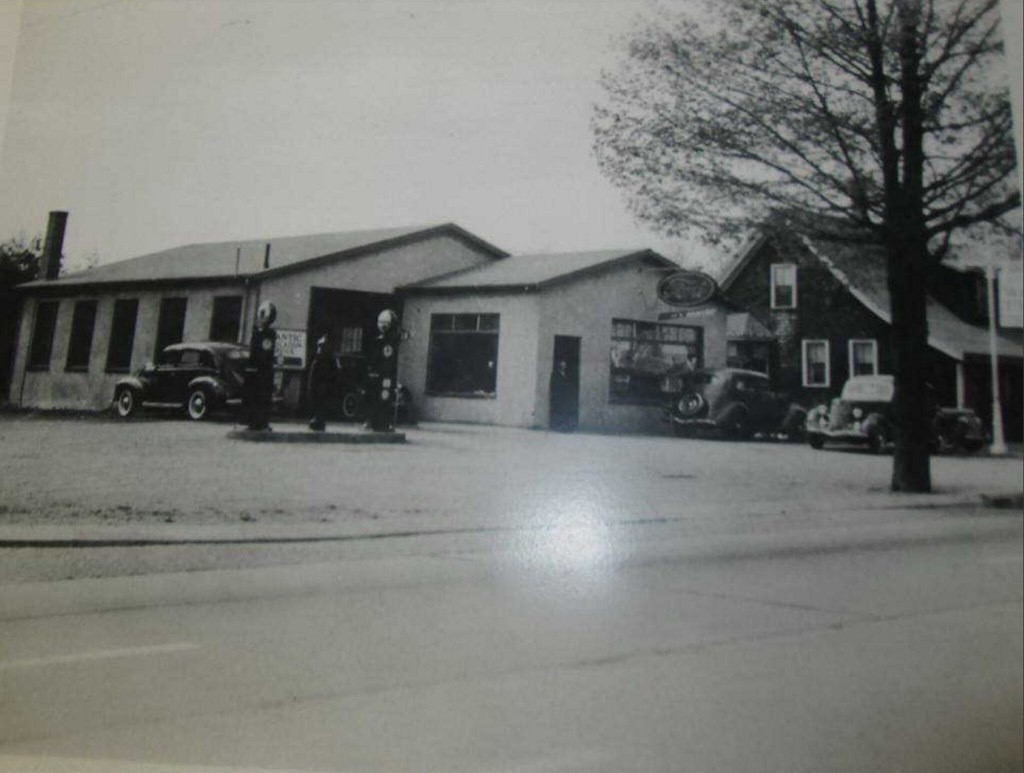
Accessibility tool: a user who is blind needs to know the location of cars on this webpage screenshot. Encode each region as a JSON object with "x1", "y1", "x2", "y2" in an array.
[
  {"x1": 663, "y1": 365, "x2": 808, "y2": 442},
  {"x1": 113, "y1": 340, "x2": 284, "y2": 423},
  {"x1": 806, "y1": 373, "x2": 983, "y2": 453}
]
